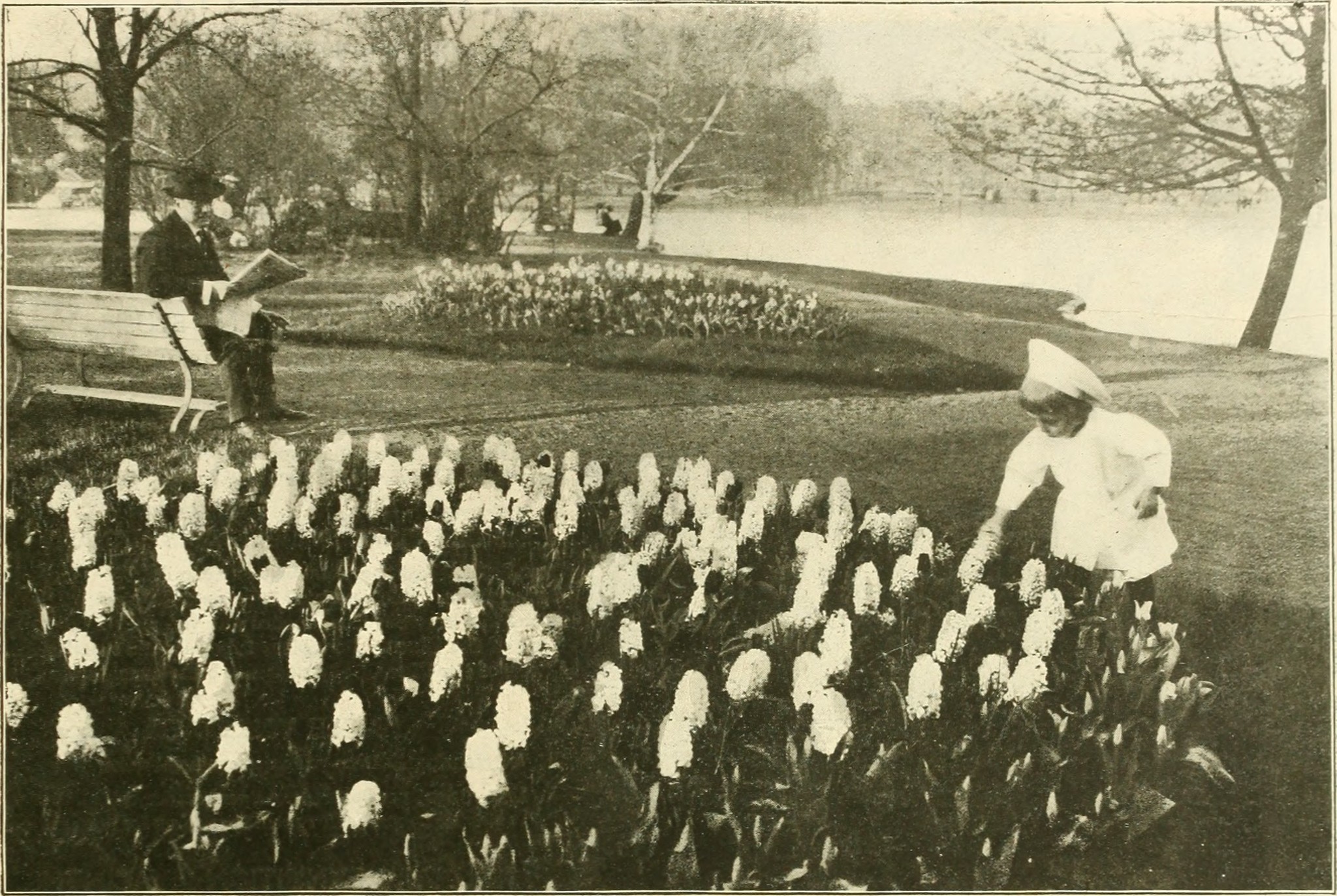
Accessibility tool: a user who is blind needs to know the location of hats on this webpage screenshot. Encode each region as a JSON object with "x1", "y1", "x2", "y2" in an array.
[
  {"x1": 163, "y1": 168, "x2": 227, "y2": 202},
  {"x1": 1026, "y1": 338, "x2": 1111, "y2": 409}
]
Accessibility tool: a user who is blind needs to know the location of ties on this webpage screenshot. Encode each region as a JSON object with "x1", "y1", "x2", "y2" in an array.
[{"x1": 196, "y1": 231, "x2": 208, "y2": 250}]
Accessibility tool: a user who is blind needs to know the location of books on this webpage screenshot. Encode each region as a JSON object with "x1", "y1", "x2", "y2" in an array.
[
  {"x1": 190, "y1": 294, "x2": 263, "y2": 338},
  {"x1": 226, "y1": 247, "x2": 307, "y2": 299}
]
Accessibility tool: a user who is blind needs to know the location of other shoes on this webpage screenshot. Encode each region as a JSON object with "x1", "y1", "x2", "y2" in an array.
[
  {"x1": 256, "y1": 399, "x2": 308, "y2": 420},
  {"x1": 236, "y1": 421, "x2": 272, "y2": 443}
]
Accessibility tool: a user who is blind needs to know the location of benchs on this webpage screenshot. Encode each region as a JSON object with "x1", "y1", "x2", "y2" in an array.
[{"x1": 5, "y1": 285, "x2": 230, "y2": 436}]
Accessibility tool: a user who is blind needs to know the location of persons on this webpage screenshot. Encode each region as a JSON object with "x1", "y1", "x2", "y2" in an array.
[
  {"x1": 595, "y1": 202, "x2": 623, "y2": 237},
  {"x1": 985, "y1": 338, "x2": 1179, "y2": 624},
  {"x1": 132, "y1": 168, "x2": 314, "y2": 439}
]
